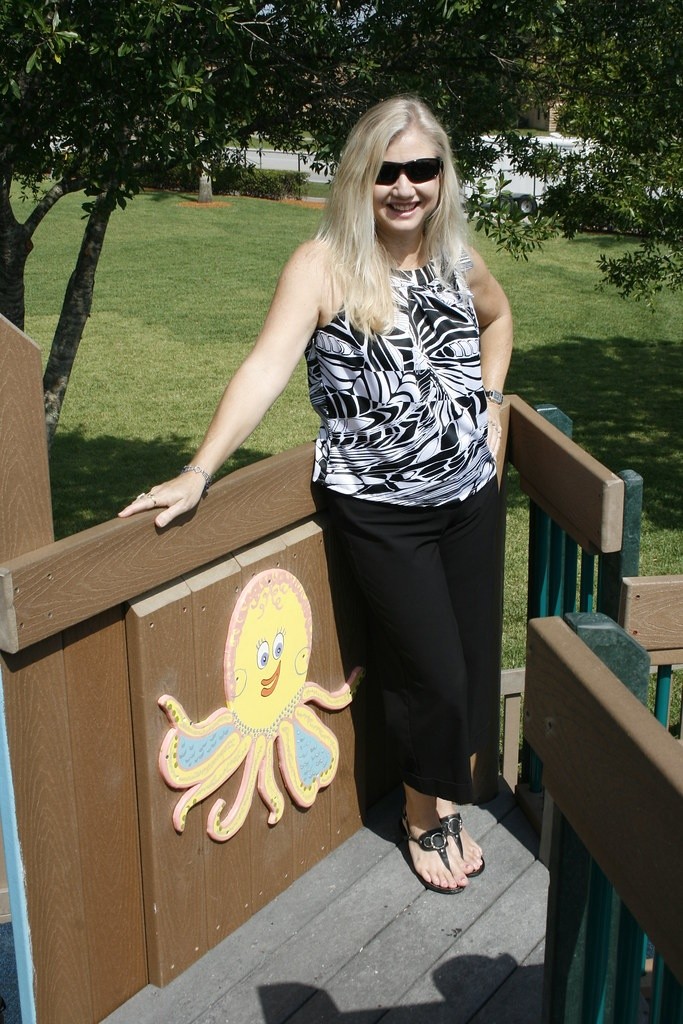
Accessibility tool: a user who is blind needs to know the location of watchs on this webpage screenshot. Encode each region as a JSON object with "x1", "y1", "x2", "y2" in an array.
[{"x1": 485, "y1": 389, "x2": 504, "y2": 404}]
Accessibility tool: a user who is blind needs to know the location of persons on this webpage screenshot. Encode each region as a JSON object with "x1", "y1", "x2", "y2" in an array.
[{"x1": 117, "y1": 92, "x2": 516, "y2": 895}]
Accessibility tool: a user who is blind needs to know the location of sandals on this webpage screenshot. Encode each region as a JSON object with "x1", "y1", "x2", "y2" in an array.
[
  {"x1": 400, "y1": 812, "x2": 468, "y2": 895},
  {"x1": 438, "y1": 814, "x2": 486, "y2": 879}
]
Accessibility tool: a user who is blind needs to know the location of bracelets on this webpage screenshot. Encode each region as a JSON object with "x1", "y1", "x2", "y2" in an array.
[{"x1": 181, "y1": 465, "x2": 212, "y2": 489}]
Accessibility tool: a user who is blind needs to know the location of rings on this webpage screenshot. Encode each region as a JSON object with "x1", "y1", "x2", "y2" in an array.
[
  {"x1": 137, "y1": 493, "x2": 145, "y2": 499},
  {"x1": 146, "y1": 493, "x2": 156, "y2": 507}
]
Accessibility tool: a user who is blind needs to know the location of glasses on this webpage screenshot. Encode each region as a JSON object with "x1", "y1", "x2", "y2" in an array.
[{"x1": 368, "y1": 156, "x2": 448, "y2": 186}]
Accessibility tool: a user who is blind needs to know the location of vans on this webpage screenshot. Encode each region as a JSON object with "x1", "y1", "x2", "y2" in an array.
[{"x1": 459, "y1": 133, "x2": 665, "y2": 217}]
